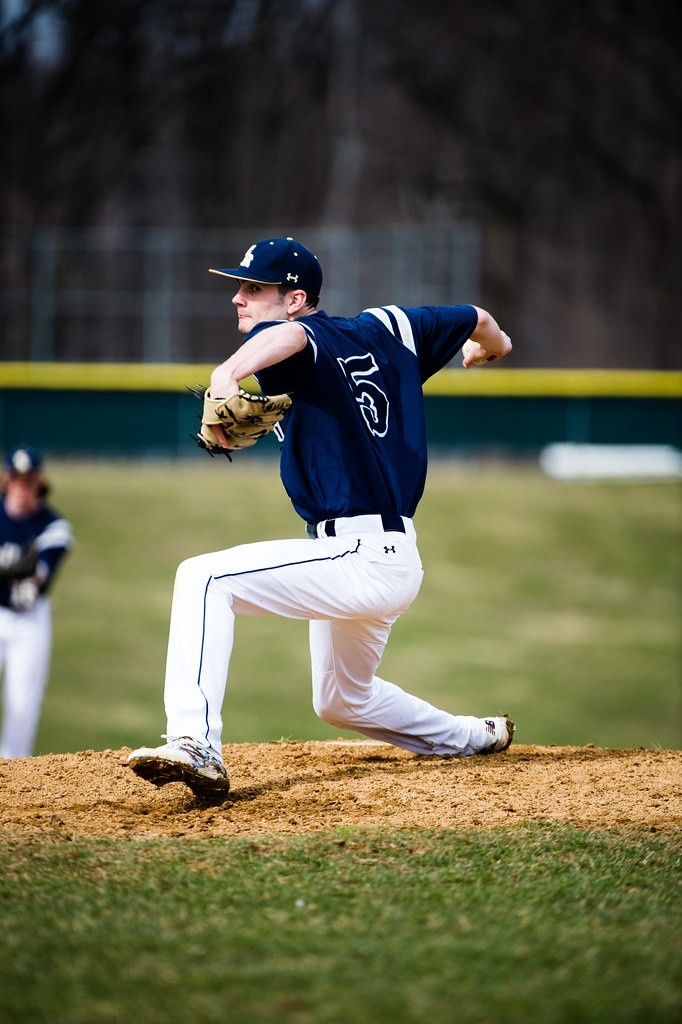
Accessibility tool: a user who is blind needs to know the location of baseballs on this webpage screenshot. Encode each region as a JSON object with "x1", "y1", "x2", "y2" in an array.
[{"x1": 463, "y1": 339, "x2": 487, "y2": 365}]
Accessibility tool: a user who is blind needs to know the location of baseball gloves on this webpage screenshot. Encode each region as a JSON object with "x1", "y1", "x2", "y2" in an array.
[{"x1": 186, "y1": 383, "x2": 293, "y2": 464}]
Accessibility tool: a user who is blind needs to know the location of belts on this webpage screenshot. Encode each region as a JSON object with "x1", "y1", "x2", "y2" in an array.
[{"x1": 305, "y1": 513, "x2": 406, "y2": 539}]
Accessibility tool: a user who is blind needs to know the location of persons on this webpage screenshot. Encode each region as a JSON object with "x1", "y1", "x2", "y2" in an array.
[
  {"x1": 124, "y1": 236, "x2": 517, "y2": 806},
  {"x1": 0, "y1": 445, "x2": 78, "y2": 759}
]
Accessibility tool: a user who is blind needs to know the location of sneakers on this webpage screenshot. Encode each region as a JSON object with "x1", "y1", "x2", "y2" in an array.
[
  {"x1": 469, "y1": 714, "x2": 516, "y2": 756},
  {"x1": 128, "y1": 736, "x2": 230, "y2": 799}
]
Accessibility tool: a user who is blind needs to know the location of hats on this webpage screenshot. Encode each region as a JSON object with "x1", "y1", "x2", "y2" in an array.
[
  {"x1": 209, "y1": 238, "x2": 323, "y2": 296},
  {"x1": 5, "y1": 446, "x2": 43, "y2": 473}
]
